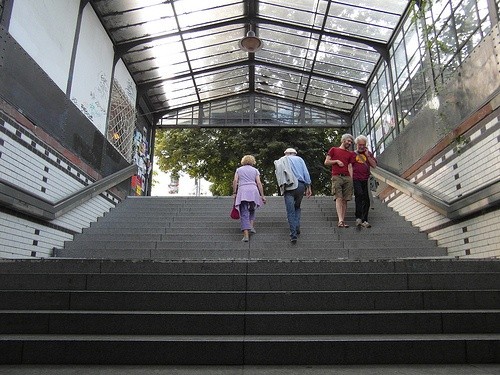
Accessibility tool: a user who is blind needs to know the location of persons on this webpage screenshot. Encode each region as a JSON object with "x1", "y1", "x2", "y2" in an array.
[
  {"x1": 233, "y1": 154, "x2": 267, "y2": 242},
  {"x1": 273, "y1": 148, "x2": 312, "y2": 242},
  {"x1": 348, "y1": 135, "x2": 378, "y2": 228},
  {"x1": 323, "y1": 133, "x2": 356, "y2": 228}
]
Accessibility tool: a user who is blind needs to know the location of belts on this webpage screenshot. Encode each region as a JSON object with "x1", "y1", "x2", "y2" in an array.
[{"x1": 340, "y1": 173, "x2": 346, "y2": 176}]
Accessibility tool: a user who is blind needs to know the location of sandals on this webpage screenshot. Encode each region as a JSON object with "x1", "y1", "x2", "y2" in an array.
[
  {"x1": 338, "y1": 221, "x2": 344, "y2": 227},
  {"x1": 344, "y1": 223, "x2": 349, "y2": 227}
]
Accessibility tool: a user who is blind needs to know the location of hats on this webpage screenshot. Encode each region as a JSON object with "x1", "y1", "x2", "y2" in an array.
[{"x1": 284, "y1": 148, "x2": 297, "y2": 154}]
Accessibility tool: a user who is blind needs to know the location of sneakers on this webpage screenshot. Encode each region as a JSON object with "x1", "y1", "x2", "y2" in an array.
[
  {"x1": 357, "y1": 222, "x2": 361, "y2": 231},
  {"x1": 362, "y1": 221, "x2": 371, "y2": 228},
  {"x1": 291, "y1": 236, "x2": 297, "y2": 241},
  {"x1": 249, "y1": 227, "x2": 256, "y2": 233},
  {"x1": 241, "y1": 236, "x2": 248, "y2": 242}
]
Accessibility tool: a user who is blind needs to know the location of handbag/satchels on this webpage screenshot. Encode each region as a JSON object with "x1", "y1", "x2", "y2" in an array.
[{"x1": 231, "y1": 208, "x2": 240, "y2": 219}]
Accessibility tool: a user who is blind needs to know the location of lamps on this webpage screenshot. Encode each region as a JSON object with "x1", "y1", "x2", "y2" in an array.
[{"x1": 238, "y1": 19, "x2": 264, "y2": 52}]
